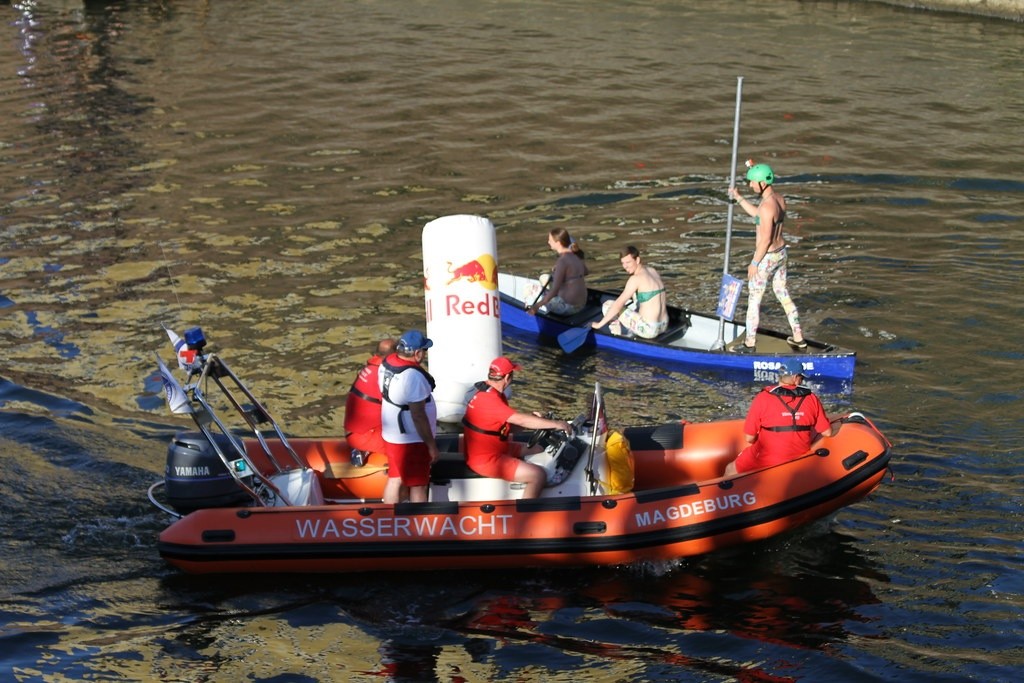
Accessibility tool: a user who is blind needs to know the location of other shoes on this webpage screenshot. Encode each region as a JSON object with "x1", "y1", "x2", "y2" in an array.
[{"x1": 349, "y1": 447, "x2": 364, "y2": 467}]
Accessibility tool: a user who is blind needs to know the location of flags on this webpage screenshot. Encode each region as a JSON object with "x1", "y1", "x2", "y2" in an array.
[
  {"x1": 157, "y1": 355, "x2": 194, "y2": 413},
  {"x1": 163, "y1": 325, "x2": 200, "y2": 374}
]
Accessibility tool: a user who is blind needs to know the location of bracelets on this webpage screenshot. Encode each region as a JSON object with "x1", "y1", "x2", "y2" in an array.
[
  {"x1": 737, "y1": 197, "x2": 743, "y2": 203},
  {"x1": 751, "y1": 260, "x2": 758, "y2": 266}
]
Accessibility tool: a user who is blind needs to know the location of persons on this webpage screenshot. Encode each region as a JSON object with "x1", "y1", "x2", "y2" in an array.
[
  {"x1": 378, "y1": 331, "x2": 439, "y2": 501},
  {"x1": 343, "y1": 338, "x2": 396, "y2": 456},
  {"x1": 585, "y1": 245, "x2": 668, "y2": 339},
  {"x1": 461, "y1": 357, "x2": 572, "y2": 499},
  {"x1": 722, "y1": 357, "x2": 832, "y2": 478},
  {"x1": 525, "y1": 228, "x2": 589, "y2": 317},
  {"x1": 728, "y1": 164, "x2": 807, "y2": 354}
]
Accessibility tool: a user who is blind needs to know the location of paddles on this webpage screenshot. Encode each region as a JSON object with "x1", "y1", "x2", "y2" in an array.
[
  {"x1": 557, "y1": 301, "x2": 631, "y2": 354},
  {"x1": 524, "y1": 271, "x2": 554, "y2": 312}
]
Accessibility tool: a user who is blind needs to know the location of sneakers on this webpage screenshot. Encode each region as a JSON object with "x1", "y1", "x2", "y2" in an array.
[
  {"x1": 786, "y1": 337, "x2": 808, "y2": 348},
  {"x1": 729, "y1": 343, "x2": 756, "y2": 354}
]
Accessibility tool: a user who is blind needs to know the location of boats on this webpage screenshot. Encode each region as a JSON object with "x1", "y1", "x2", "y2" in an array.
[
  {"x1": 498, "y1": 272, "x2": 857, "y2": 382},
  {"x1": 147, "y1": 412, "x2": 895, "y2": 554}
]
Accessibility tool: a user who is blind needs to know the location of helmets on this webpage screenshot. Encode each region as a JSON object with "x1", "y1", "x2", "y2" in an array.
[{"x1": 747, "y1": 163, "x2": 774, "y2": 184}]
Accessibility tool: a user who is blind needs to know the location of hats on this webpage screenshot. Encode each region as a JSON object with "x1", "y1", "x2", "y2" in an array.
[
  {"x1": 779, "y1": 358, "x2": 812, "y2": 379},
  {"x1": 489, "y1": 357, "x2": 522, "y2": 378},
  {"x1": 397, "y1": 329, "x2": 433, "y2": 353}
]
